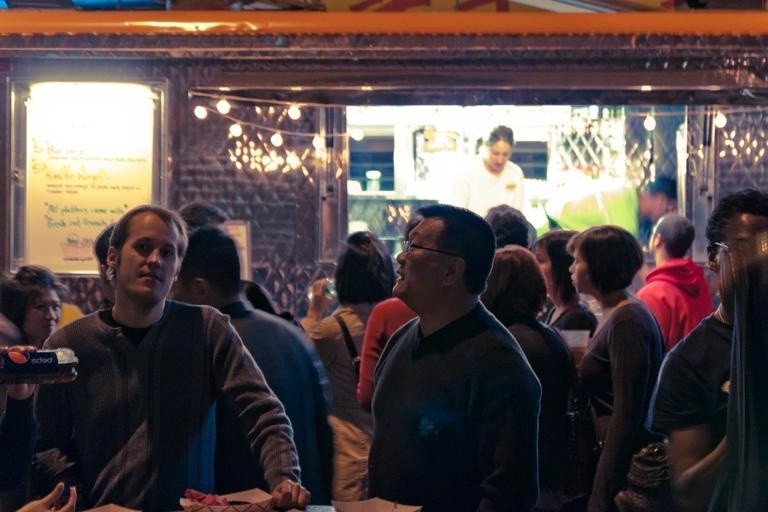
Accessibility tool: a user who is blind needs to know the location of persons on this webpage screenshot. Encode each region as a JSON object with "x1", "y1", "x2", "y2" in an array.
[
  {"x1": 169, "y1": 225, "x2": 331, "y2": 505},
  {"x1": 643, "y1": 188, "x2": 766, "y2": 512},
  {"x1": 566, "y1": 227, "x2": 664, "y2": 512},
  {"x1": 1, "y1": 345, "x2": 79, "y2": 512},
  {"x1": 364, "y1": 206, "x2": 543, "y2": 511},
  {"x1": 640, "y1": 215, "x2": 713, "y2": 350},
  {"x1": 3, "y1": 125, "x2": 768, "y2": 512},
  {"x1": 2, "y1": 265, "x2": 62, "y2": 349},
  {"x1": 32, "y1": 205, "x2": 311, "y2": 507},
  {"x1": 299, "y1": 231, "x2": 417, "y2": 512}
]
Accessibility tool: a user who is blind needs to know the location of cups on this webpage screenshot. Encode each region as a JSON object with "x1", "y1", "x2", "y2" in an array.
[{"x1": 559, "y1": 329, "x2": 589, "y2": 368}]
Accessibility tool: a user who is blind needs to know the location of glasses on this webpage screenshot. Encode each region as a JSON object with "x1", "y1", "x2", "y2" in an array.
[{"x1": 400, "y1": 239, "x2": 466, "y2": 261}]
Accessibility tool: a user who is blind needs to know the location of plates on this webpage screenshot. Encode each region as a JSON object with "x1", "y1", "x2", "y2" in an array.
[
  {"x1": 180, "y1": 487, "x2": 273, "y2": 511},
  {"x1": 331, "y1": 497, "x2": 422, "y2": 512}
]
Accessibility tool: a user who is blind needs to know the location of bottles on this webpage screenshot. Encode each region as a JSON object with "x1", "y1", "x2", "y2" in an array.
[
  {"x1": 566, "y1": 392, "x2": 580, "y2": 425},
  {"x1": 0, "y1": 347, "x2": 79, "y2": 386},
  {"x1": 365, "y1": 170, "x2": 381, "y2": 192},
  {"x1": 306, "y1": 278, "x2": 337, "y2": 299}
]
